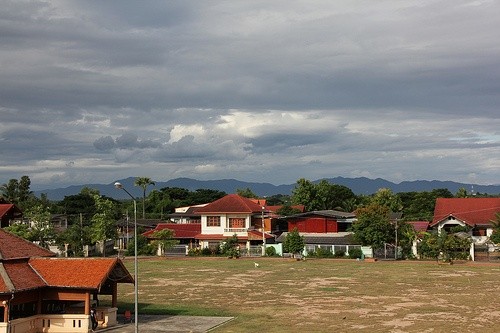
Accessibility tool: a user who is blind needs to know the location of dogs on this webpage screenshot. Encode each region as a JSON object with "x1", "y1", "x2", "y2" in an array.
[{"x1": 253, "y1": 262, "x2": 259, "y2": 268}]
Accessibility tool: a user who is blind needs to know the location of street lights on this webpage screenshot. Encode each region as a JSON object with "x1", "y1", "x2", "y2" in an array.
[{"x1": 114, "y1": 182, "x2": 139, "y2": 333}]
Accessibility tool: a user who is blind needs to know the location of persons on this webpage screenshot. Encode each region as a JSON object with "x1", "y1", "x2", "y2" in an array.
[{"x1": 90, "y1": 303, "x2": 99, "y2": 333}]
